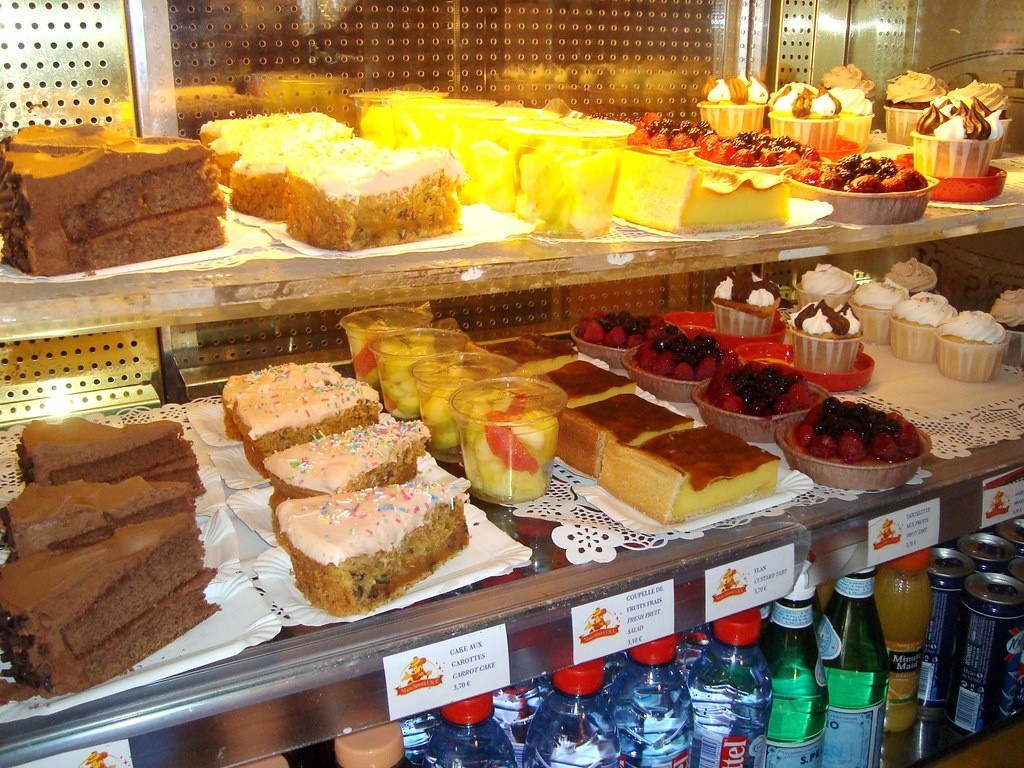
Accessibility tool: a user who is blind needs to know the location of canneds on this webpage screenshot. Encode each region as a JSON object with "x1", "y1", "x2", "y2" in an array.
[{"x1": 913, "y1": 517, "x2": 1024, "y2": 736}]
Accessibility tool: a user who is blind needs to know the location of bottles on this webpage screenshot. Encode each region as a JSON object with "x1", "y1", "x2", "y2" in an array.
[{"x1": 227, "y1": 521, "x2": 929, "y2": 768}]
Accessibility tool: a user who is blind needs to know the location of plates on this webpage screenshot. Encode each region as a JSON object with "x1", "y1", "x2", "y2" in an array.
[
  {"x1": 572, "y1": 311, "x2": 932, "y2": 490},
  {"x1": 648, "y1": 147, "x2": 1007, "y2": 225}
]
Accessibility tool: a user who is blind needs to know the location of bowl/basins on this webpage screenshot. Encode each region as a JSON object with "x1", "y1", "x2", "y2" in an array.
[
  {"x1": 697, "y1": 104, "x2": 1012, "y2": 178},
  {"x1": 710, "y1": 284, "x2": 1024, "y2": 382}
]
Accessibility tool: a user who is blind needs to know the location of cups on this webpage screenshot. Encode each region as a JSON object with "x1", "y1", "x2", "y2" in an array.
[
  {"x1": 352, "y1": 88, "x2": 636, "y2": 235},
  {"x1": 449, "y1": 377, "x2": 568, "y2": 504},
  {"x1": 368, "y1": 328, "x2": 464, "y2": 422},
  {"x1": 341, "y1": 306, "x2": 436, "y2": 392},
  {"x1": 408, "y1": 353, "x2": 520, "y2": 464}
]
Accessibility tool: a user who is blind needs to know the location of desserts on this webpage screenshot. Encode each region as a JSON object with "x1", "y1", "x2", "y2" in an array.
[
  {"x1": 0, "y1": 64, "x2": 1012, "y2": 274},
  {"x1": 0, "y1": 254, "x2": 1024, "y2": 708}
]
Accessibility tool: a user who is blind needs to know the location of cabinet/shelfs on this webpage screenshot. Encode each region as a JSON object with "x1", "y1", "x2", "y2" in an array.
[{"x1": 2, "y1": 1, "x2": 1024, "y2": 768}]
